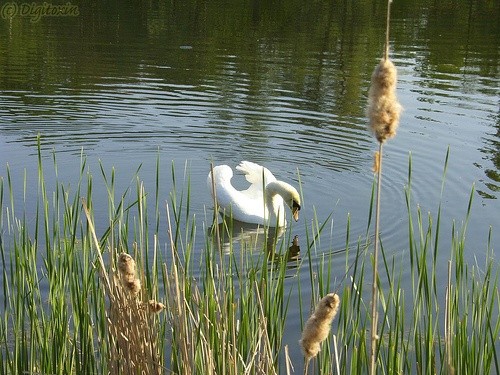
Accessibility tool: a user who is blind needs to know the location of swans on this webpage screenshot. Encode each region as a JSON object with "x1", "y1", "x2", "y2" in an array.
[{"x1": 206, "y1": 161, "x2": 301, "y2": 228}]
